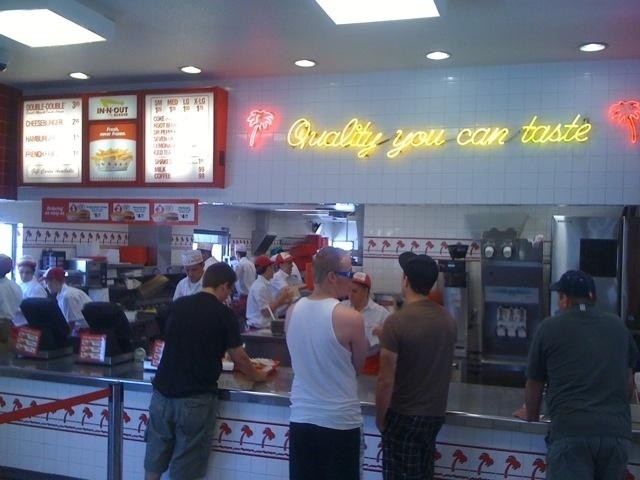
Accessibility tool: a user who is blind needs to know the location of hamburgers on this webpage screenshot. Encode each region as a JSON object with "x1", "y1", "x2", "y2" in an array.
[
  {"x1": 79, "y1": 210, "x2": 90, "y2": 218},
  {"x1": 164, "y1": 212, "x2": 179, "y2": 223},
  {"x1": 122, "y1": 211, "x2": 135, "y2": 222}
]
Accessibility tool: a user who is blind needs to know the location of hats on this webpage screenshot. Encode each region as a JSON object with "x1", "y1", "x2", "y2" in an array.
[
  {"x1": 277, "y1": 252, "x2": 295, "y2": 264},
  {"x1": 549, "y1": 270, "x2": 597, "y2": 293},
  {"x1": 0, "y1": 253, "x2": 13, "y2": 273},
  {"x1": 16, "y1": 255, "x2": 36, "y2": 267},
  {"x1": 399, "y1": 250, "x2": 439, "y2": 294},
  {"x1": 234, "y1": 243, "x2": 247, "y2": 253},
  {"x1": 197, "y1": 241, "x2": 214, "y2": 251},
  {"x1": 181, "y1": 249, "x2": 204, "y2": 265},
  {"x1": 350, "y1": 272, "x2": 371, "y2": 288},
  {"x1": 39, "y1": 268, "x2": 65, "y2": 282},
  {"x1": 255, "y1": 256, "x2": 275, "y2": 271}
]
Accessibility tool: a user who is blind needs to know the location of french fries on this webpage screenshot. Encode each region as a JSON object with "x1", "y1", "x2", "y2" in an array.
[{"x1": 93, "y1": 148, "x2": 134, "y2": 164}]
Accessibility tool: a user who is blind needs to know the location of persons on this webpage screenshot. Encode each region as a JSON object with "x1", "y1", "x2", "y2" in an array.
[
  {"x1": 512, "y1": 268, "x2": 637, "y2": 480},
  {"x1": 17, "y1": 255, "x2": 48, "y2": 306},
  {"x1": 35, "y1": 265, "x2": 95, "y2": 337},
  {"x1": 342, "y1": 270, "x2": 389, "y2": 378},
  {"x1": 141, "y1": 262, "x2": 268, "y2": 479},
  {"x1": 0, "y1": 253, "x2": 23, "y2": 346},
  {"x1": 282, "y1": 245, "x2": 370, "y2": 479},
  {"x1": 173, "y1": 239, "x2": 304, "y2": 332},
  {"x1": 374, "y1": 249, "x2": 457, "y2": 480}
]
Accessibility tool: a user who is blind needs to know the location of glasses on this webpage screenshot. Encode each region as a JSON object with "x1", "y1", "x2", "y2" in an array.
[{"x1": 336, "y1": 270, "x2": 354, "y2": 279}]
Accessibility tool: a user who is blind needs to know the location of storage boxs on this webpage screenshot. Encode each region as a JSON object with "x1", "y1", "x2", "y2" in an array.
[{"x1": 119, "y1": 246, "x2": 156, "y2": 265}]
